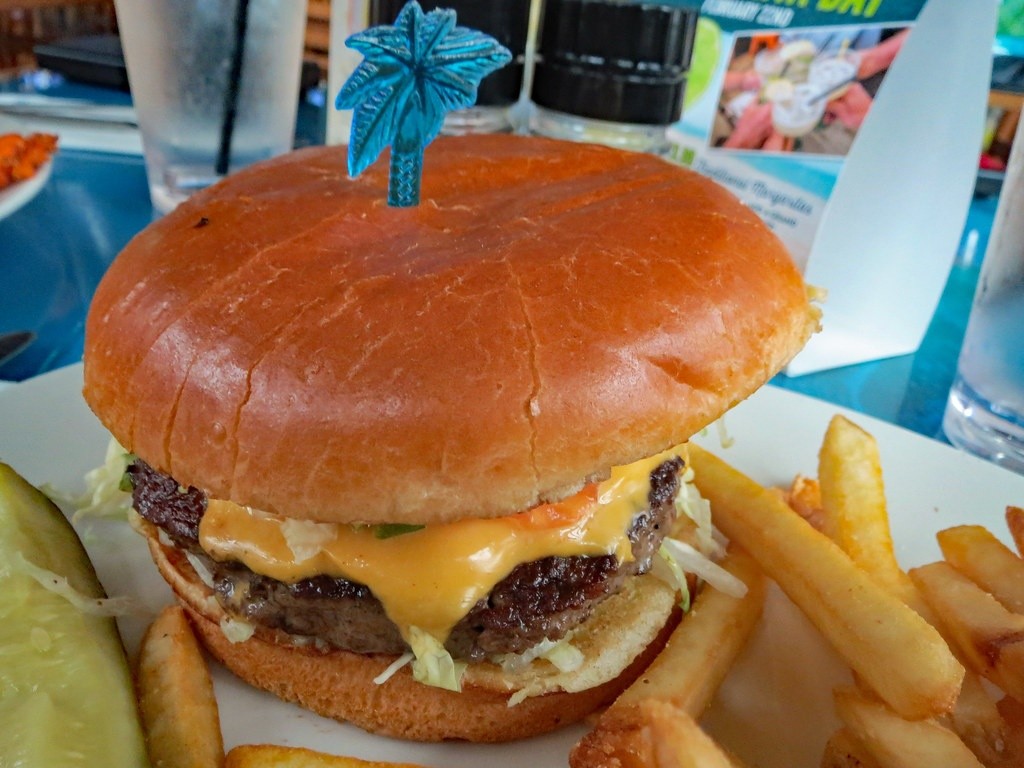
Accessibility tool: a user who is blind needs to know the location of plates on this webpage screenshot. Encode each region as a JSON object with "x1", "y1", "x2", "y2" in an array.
[
  {"x1": 0, "y1": 117, "x2": 54, "y2": 215},
  {"x1": 0, "y1": 361, "x2": 1024, "y2": 768}
]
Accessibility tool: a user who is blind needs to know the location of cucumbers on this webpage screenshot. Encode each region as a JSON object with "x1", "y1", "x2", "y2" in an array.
[{"x1": 0, "y1": 462, "x2": 151, "y2": 768}]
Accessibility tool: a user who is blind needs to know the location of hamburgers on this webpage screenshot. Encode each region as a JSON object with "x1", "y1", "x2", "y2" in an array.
[{"x1": 81, "y1": 135, "x2": 824, "y2": 744}]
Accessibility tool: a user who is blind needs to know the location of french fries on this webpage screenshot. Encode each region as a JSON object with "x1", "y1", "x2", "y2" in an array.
[{"x1": 136, "y1": 415, "x2": 1023, "y2": 767}]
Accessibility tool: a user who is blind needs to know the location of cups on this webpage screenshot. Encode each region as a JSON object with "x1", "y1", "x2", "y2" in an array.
[
  {"x1": 114, "y1": 2, "x2": 308, "y2": 222},
  {"x1": 942, "y1": 99, "x2": 1024, "y2": 473},
  {"x1": 809, "y1": 48, "x2": 864, "y2": 102},
  {"x1": 770, "y1": 84, "x2": 829, "y2": 137}
]
